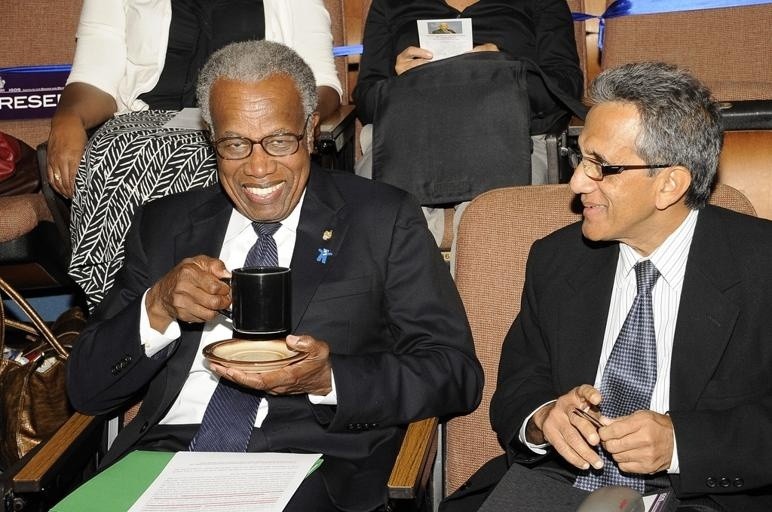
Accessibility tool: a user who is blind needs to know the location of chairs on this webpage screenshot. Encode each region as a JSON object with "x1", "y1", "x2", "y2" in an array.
[{"x1": 0, "y1": 0, "x2": 772, "y2": 512}]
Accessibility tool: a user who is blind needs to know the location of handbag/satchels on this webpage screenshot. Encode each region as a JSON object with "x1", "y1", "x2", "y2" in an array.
[{"x1": 0, "y1": 278, "x2": 80, "y2": 469}]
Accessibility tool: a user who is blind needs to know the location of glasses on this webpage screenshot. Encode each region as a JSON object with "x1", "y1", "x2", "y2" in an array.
[
  {"x1": 568, "y1": 151, "x2": 684, "y2": 183},
  {"x1": 209, "y1": 112, "x2": 313, "y2": 161}
]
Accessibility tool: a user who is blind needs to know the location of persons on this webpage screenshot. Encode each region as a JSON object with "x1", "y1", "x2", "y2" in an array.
[
  {"x1": 352, "y1": 1, "x2": 586, "y2": 283},
  {"x1": 437, "y1": 59, "x2": 772, "y2": 511},
  {"x1": 65, "y1": 38, "x2": 484, "y2": 511},
  {"x1": 430, "y1": 22, "x2": 455, "y2": 34},
  {"x1": 42, "y1": 1, "x2": 349, "y2": 327}
]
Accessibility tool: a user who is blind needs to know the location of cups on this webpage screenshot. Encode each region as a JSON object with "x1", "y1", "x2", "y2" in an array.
[{"x1": 214, "y1": 265, "x2": 293, "y2": 336}]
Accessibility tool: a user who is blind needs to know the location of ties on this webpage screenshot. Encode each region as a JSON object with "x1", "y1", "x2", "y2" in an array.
[
  {"x1": 570, "y1": 259, "x2": 663, "y2": 496},
  {"x1": 189, "y1": 218, "x2": 282, "y2": 452}
]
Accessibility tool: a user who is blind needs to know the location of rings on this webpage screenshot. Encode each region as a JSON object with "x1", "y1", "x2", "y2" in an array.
[{"x1": 53, "y1": 171, "x2": 62, "y2": 182}]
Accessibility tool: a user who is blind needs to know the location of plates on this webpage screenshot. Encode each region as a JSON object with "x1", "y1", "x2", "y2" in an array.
[{"x1": 201, "y1": 338, "x2": 309, "y2": 376}]
